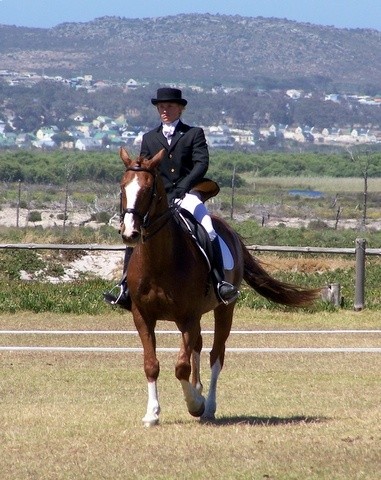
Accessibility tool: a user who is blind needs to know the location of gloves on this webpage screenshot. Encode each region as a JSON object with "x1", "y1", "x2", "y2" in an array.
[{"x1": 170, "y1": 188, "x2": 186, "y2": 198}]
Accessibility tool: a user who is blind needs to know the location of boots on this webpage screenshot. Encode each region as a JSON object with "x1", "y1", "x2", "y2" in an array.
[
  {"x1": 102, "y1": 247, "x2": 133, "y2": 313},
  {"x1": 209, "y1": 236, "x2": 234, "y2": 300}
]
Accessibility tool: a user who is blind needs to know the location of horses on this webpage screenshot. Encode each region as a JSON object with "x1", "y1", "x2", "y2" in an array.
[{"x1": 113, "y1": 144, "x2": 339, "y2": 430}]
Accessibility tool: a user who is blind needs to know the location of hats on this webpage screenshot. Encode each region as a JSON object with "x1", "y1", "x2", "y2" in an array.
[{"x1": 150, "y1": 87, "x2": 188, "y2": 106}]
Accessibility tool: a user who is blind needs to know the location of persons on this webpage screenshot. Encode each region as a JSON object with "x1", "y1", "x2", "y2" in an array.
[{"x1": 103, "y1": 88, "x2": 238, "y2": 311}]
